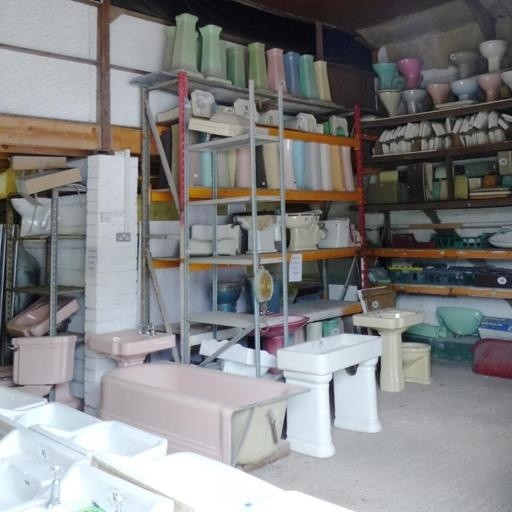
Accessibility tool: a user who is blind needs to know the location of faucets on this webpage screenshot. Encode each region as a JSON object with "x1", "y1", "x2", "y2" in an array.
[{"x1": 141, "y1": 325, "x2": 151, "y2": 336}]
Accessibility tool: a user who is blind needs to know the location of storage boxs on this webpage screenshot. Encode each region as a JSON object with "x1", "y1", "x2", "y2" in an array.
[{"x1": 10, "y1": 154, "x2": 82, "y2": 194}]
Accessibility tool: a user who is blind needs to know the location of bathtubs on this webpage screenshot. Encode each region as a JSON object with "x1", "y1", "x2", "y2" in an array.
[{"x1": 102, "y1": 361, "x2": 310, "y2": 469}]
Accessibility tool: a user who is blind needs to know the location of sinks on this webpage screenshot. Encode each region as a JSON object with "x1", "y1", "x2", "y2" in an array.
[
  {"x1": 277, "y1": 334, "x2": 384, "y2": 375},
  {"x1": 84, "y1": 330, "x2": 175, "y2": 355},
  {"x1": 0, "y1": 381, "x2": 354, "y2": 512},
  {"x1": 6, "y1": 295, "x2": 80, "y2": 337},
  {"x1": 353, "y1": 308, "x2": 423, "y2": 329}
]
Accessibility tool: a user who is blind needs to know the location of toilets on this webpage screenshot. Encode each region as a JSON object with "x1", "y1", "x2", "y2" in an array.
[{"x1": 0, "y1": 337, "x2": 77, "y2": 399}]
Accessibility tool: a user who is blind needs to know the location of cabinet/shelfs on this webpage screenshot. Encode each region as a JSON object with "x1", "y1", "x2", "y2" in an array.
[
  {"x1": 356, "y1": 97, "x2": 512, "y2": 299},
  {"x1": 136, "y1": 69, "x2": 369, "y2": 378},
  {"x1": 4, "y1": 183, "x2": 88, "y2": 401}
]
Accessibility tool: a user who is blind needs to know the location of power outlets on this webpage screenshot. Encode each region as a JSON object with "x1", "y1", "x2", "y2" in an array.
[{"x1": 116, "y1": 233, "x2": 130, "y2": 242}]
